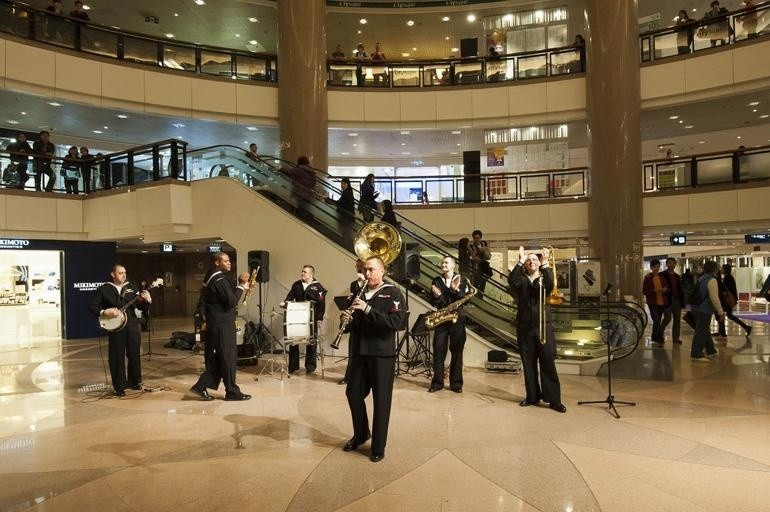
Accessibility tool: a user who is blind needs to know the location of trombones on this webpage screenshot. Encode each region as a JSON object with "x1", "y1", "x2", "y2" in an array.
[{"x1": 539, "y1": 245, "x2": 564, "y2": 345}]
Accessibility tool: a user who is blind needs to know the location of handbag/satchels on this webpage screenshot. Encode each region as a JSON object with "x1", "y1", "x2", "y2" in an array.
[
  {"x1": 682, "y1": 310, "x2": 696, "y2": 330},
  {"x1": 721, "y1": 281, "x2": 736, "y2": 307}
]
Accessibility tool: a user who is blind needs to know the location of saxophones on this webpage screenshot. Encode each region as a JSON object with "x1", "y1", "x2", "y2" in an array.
[{"x1": 425, "y1": 277, "x2": 478, "y2": 328}]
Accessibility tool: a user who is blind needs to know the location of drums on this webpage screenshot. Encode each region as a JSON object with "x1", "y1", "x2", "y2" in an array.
[{"x1": 283, "y1": 301, "x2": 317, "y2": 344}]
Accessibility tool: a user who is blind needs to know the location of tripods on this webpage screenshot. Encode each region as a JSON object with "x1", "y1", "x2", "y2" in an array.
[
  {"x1": 395, "y1": 282, "x2": 433, "y2": 378},
  {"x1": 245, "y1": 283, "x2": 289, "y2": 357},
  {"x1": 255, "y1": 315, "x2": 291, "y2": 382},
  {"x1": 139, "y1": 306, "x2": 169, "y2": 362},
  {"x1": 577, "y1": 293, "x2": 637, "y2": 419}
]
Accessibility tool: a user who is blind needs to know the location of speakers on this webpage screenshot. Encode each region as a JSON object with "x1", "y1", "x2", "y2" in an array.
[
  {"x1": 392, "y1": 240, "x2": 421, "y2": 283},
  {"x1": 247, "y1": 249, "x2": 270, "y2": 284}
]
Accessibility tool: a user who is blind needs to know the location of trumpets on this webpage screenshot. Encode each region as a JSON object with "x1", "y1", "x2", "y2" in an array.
[{"x1": 240, "y1": 266, "x2": 260, "y2": 306}]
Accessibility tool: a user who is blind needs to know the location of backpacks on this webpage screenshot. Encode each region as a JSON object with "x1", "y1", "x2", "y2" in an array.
[{"x1": 687, "y1": 275, "x2": 716, "y2": 305}]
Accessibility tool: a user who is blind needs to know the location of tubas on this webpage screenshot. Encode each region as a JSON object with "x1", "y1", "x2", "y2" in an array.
[{"x1": 354, "y1": 222, "x2": 402, "y2": 282}]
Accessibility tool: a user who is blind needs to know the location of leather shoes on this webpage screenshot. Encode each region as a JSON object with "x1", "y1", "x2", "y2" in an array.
[
  {"x1": 337, "y1": 378, "x2": 346, "y2": 385},
  {"x1": 370, "y1": 451, "x2": 385, "y2": 461},
  {"x1": 343, "y1": 429, "x2": 371, "y2": 452},
  {"x1": 224, "y1": 392, "x2": 252, "y2": 401},
  {"x1": 428, "y1": 384, "x2": 444, "y2": 393},
  {"x1": 450, "y1": 386, "x2": 462, "y2": 393},
  {"x1": 519, "y1": 397, "x2": 541, "y2": 407},
  {"x1": 191, "y1": 384, "x2": 214, "y2": 400},
  {"x1": 548, "y1": 401, "x2": 566, "y2": 413}
]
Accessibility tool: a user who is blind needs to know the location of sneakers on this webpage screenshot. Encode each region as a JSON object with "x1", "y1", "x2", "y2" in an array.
[{"x1": 745, "y1": 325, "x2": 752, "y2": 337}]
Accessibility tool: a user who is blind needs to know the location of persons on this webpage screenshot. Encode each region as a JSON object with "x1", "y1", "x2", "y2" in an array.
[
  {"x1": 339, "y1": 256, "x2": 407, "y2": 462},
  {"x1": 642, "y1": 260, "x2": 670, "y2": 347},
  {"x1": 351, "y1": 43, "x2": 370, "y2": 86},
  {"x1": 69, "y1": 0, "x2": 90, "y2": 19},
  {"x1": 738, "y1": 0, "x2": 757, "y2": 38},
  {"x1": 646, "y1": 257, "x2": 682, "y2": 344},
  {"x1": 677, "y1": 10, "x2": 698, "y2": 55},
  {"x1": 359, "y1": 173, "x2": 380, "y2": 225},
  {"x1": 80, "y1": 147, "x2": 93, "y2": 193},
  {"x1": 704, "y1": 1, "x2": 733, "y2": 46},
  {"x1": 245, "y1": 143, "x2": 259, "y2": 186},
  {"x1": 273, "y1": 156, "x2": 317, "y2": 202},
  {"x1": 320, "y1": 177, "x2": 355, "y2": 250},
  {"x1": 190, "y1": 252, "x2": 251, "y2": 401},
  {"x1": 470, "y1": 230, "x2": 485, "y2": 299},
  {"x1": 458, "y1": 238, "x2": 473, "y2": 284},
  {"x1": 199, "y1": 287, "x2": 206, "y2": 324},
  {"x1": 573, "y1": 34, "x2": 585, "y2": 71},
  {"x1": 328, "y1": 44, "x2": 347, "y2": 84},
  {"x1": 685, "y1": 265, "x2": 702, "y2": 312},
  {"x1": 338, "y1": 259, "x2": 366, "y2": 385},
  {"x1": 60, "y1": 146, "x2": 80, "y2": 194},
  {"x1": 714, "y1": 269, "x2": 726, "y2": 340},
  {"x1": 6, "y1": 133, "x2": 33, "y2": 190},
  {"x1": 88, "y1": 264, "x2": 152, "y2": 397},
  {"x1": 279, "y1": 265, "x2": 328, "y2": 374},
  {"x1": 381, "y1": 200, "x2": 403, "y2": 282},
  {"x1": 429, "y1": 256, "x2": 470, "y2": 393},
  {"x1": 33, "y1": 131, "x2": 57, "y2": 192},
  {"x1": 507, "y1": 245, "x2": 566, "y2": 413},
  {"x1": 44, "y1": 0, "x2": 64, "y2": 16},
  {"x1": 689, "y1": 260, "x2": 725, "y2": 362},
  {"x1": 487, "y1": 47, "x2": 500, "y2": 82},
  {"x1": 709, "y1": 264, "x2": 752, "y2": 338},
  {"x1": 370, "y1": 42, "x2": 387, "y2": 86}
]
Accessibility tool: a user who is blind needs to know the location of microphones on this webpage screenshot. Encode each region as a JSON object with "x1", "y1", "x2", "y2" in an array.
[
  {"x1": 603, "y1": 283, "x2": 613, "y2": 296},
  {"x1": 133, "y1": 280, "x2": 147, "y2": 294}
]
